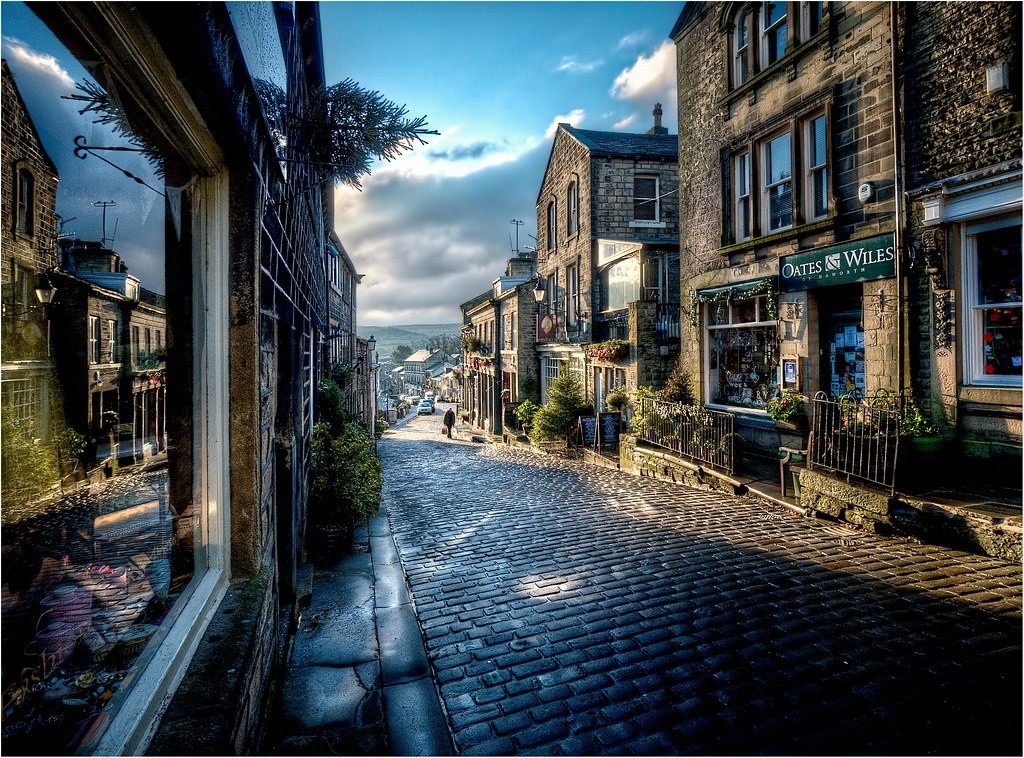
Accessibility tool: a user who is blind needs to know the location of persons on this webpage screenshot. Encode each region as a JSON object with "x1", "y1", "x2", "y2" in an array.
[{"x1": 444, "y1": 407, "x2": 456, "y2": 439}]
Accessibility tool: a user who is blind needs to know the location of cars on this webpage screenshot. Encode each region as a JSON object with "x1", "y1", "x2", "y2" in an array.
[
  {"x1": 425, "y1": 394, "x2": 434, "y2": 401},
  {"x1": 437, "y1": 395, "x2": 446, "y2": 402},
  {"x1": 415, "y1": 402, "x2": 433, "y2": 415},
  {"x1": 419, "y1": 399, "x2": 436, "y2": 413},
  {"x1": 412, "y1": 397, "x2": 419, "y2": 405}
]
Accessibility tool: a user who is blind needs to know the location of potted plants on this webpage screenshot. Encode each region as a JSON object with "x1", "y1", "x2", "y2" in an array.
[
  {"x1": 306, "y1": 421, "x2": 382, "y2": 556},
  {"x1": 898, "y1": 400, "x2": 944, "y2": 452},
  {"x1": 667, "y1": 423, "x2": 747, "y2": 469}
]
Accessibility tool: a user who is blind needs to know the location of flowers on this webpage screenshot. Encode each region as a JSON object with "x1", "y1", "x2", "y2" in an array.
[{"x1": 767, "y1": 390, "x2": 806, "y2": 422}]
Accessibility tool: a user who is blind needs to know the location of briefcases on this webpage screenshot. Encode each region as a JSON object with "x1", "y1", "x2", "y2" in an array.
[{"x1": 441, "y1": 425, "x2": 448, "y2": 434}]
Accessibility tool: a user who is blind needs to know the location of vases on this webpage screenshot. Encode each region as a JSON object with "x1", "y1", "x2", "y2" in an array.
[{"x1": 776, "y1": 419, "x2": 801, "y2": 430}]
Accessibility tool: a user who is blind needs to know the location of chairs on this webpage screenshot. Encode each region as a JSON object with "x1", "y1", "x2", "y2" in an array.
[{"x1": 779, "y1": 431, "x2": 832, "y2": 497}]
